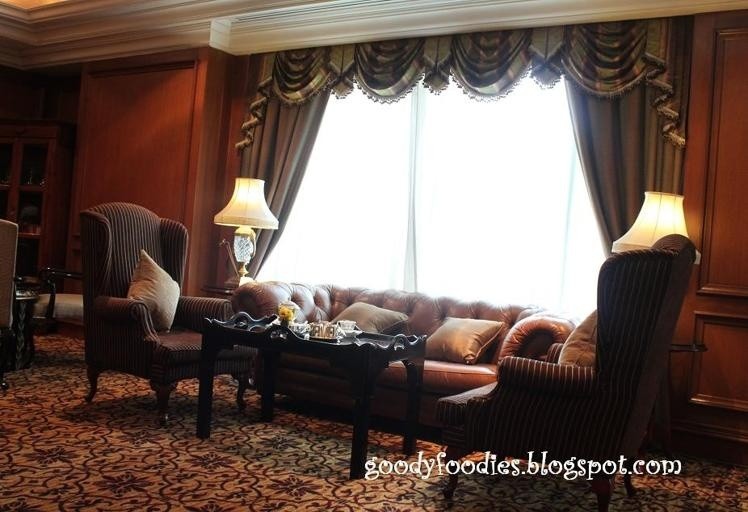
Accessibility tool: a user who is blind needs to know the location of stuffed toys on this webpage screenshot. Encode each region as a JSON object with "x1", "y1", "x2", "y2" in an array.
[{"x1": 276, "y1": 300, "x2": 298, "y2": 322}]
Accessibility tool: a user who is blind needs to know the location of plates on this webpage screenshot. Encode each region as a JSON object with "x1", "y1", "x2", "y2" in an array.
[{"x1": 340, "y1": 331, "x2": 364, "y2": 338}]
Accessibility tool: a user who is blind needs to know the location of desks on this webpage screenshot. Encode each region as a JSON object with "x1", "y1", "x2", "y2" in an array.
[
  {"x1": 666, "y1": 336, "x2": 707, "y2": 393},
  {"x1": 7, "y1": 288, "x2": 38, "y2": 371}
]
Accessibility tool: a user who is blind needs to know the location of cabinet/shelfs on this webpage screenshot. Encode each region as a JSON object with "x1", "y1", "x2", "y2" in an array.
[
  {"x1": 198, "y1": 283, "x2": 245, "y2": 299},
  {"x1": 0, "y1": 121, "x2": 73, "y2": 283}
]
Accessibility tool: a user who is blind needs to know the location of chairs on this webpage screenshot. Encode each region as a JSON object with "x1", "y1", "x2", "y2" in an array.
[
  {"x1": 434, "y1": 235, "x2": 697, "y2": 512},
  {"x1": 33, "y1": 267, "x2": 87, "y2": 322},
  {"x1": 0, "y1": 219, "x2": 19, "y2": 393},
  {"x1": 79, "y1": 201, "x2": 251, "y2": 417}
]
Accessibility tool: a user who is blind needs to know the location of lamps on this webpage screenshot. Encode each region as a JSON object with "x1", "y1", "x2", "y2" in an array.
[
  {"x1": 212, "y1": 177, "x2": 280, "y2": 287},
  {"x1": 611, "y1": 191, "x2": 702, "y2": 265}
]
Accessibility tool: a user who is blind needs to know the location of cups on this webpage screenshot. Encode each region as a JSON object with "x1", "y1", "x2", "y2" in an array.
[
  {"x1": 309, "y1": 322, "x2": 335, "y2": 338},
  {"x1": 337, "y1": 318, "x2": 355, "y2": 331}
]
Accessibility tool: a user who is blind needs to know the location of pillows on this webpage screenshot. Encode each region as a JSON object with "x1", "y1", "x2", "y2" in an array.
[
  {"x1": 423, "y1": 315, "x2": 504, "y2": 365},
  {"x1": 558, "y1": 309, "x2": 597, "y2": 368},
  {"x1": 127, "y1": 250, "x2": 181, "y2": 332},
  {"x1": 331, "y1": 302, "x2": 409, "y2": 338}
]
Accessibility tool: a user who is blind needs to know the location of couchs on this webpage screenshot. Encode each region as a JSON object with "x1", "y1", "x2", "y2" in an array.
[{"x1": 230, "y1": 281, "x2": 574, "y2": 439}]
